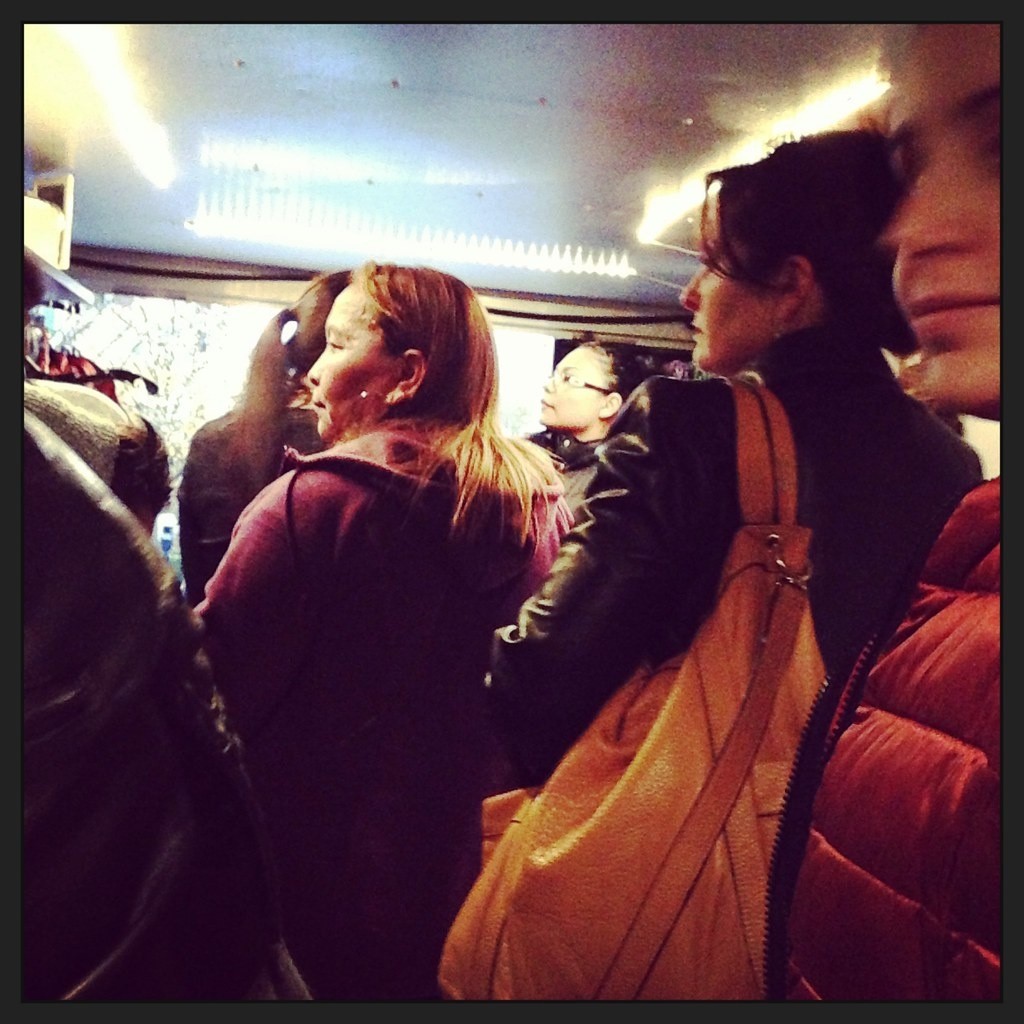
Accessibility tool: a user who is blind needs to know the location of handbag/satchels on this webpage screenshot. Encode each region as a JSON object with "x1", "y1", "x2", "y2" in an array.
[{"x1": 438, "y1": 377, "x2": 825, "y2": 1000}]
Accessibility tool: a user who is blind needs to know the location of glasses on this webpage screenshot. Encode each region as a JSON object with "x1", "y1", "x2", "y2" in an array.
[{"x1": 550, "y1": 370, "x2": 611, "y2": 395}]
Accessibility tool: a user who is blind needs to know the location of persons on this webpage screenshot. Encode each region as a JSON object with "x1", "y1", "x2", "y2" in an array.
[
  {"x1": 175, "y1": 267, "x2": 357, "y2": 614},
  {"x1": 761, "y1": 24, "x2": 1001, "y2": 1005},
  {"x1": 518, "y1": 328, "x2": 653, "y2": 511},
  {"x1": 21, "y1": 410, "x2": 194, "y2": 1002},
  {"x1": 489, "y1": 127, "x2": 986, "y2": 999},
  {"x1": 194, "y1": 263, "x2": 579, "y2": 999}
]
